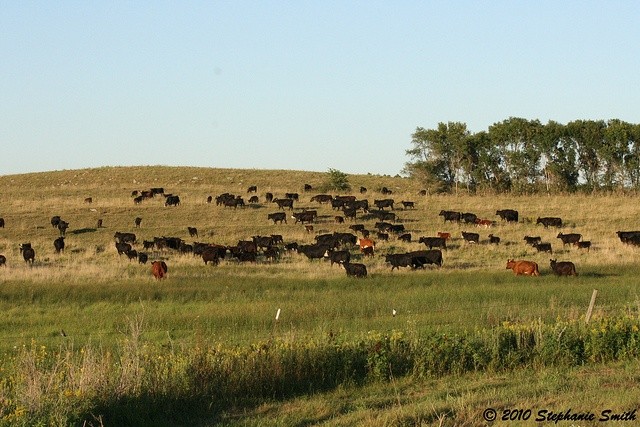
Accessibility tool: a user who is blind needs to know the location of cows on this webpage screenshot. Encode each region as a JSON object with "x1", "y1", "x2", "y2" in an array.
[
  {"x1": 361, "y1": 229, "x2": 370, "y2": 237},
  {"x1": 98, "y1": 218, "x2": 103, "y2": 227},
  {"x1": 19, "y1": 243, "x2": 32, "y2": 254},
  {"x1": 496, "y1": 209, "x2": 514, "y2": 222},
  {"x1": 310, "y1": 195, "x2": 334, "y2": 204},
  {"x1": 85, "y1": 197, "x2": 92, "y2": 203},
  {"x1": 154, "y1": 232, "x2": 192, "y2": 258},
  {"x1": 51, "y1": 216, "x2": 62, "y2": 227},
  {"x1": 114, "y1": 231, "x2": 136, "y2": 244},
  {"x1": 377, "y1": 232, "x2": 388, "y2": 240},
  {"x1": 615, "y1": 231, "x2": 640, "y2": 246},
  {"x1": 268, "y1": 212, "x2": 288, "y2": 223},
  {"x1": 272, "y1": 198, "x2": 294, "y2": 211},
  {"x1": 266, "y1": 192, "x2": 273, "y2": 201},
  {"x1": 461, "y1": 213, "x2": 477, "y2": 226},
  {"x1": 339, "y1": 260, "x2": 366, "y2": 278},
  {"x1": 374, "y1": 199, "x2": 398, "y2": 210},
  {"x1": 536, "y1": 216, "x2": 562, "y2": 229},
  {"x1": 439, "y1": 210, "x2": 461, "y2": 224},
  {"x1": 142, "y1": 240, "x2": 154, "y2": 251},
  {"x1": 502, "y1": 211, "x2": 521, "y2": 224},
  {"x1": 524, "y1": 236, "x2": 542, "y2": 246},
  {"x1": 386, "y1": 253, "x2": 416, "y2": 271},
  {"x1": 215, "y1": 192, "x2": 246, "y2": 210},
  {"x1": 304, "y1": 224, "x2": 314, "y2": 233},
  {"x1": 327, "y1": 248, "x2": 350, "y2": 269},
  {"x1": 549, "y1": 258, "x2": 578, "y2": 277},
  {"x1": 335, "y1": 196, "x2": 356, "y2": 204},
  {"x1": 247, "y1": 186, "x2": 256, "y2": 192},
  {"x1": 331, "y1": 199, "x2": 347, "y2": 210},
  {"x1": 314, "y1": 234, "x2": 336, "y2": 242},
  {"x1": 135, "y1": 217, "x2": 142, "y2": 229},
  {"x1": 298, "y1": 243, "x2": 316, "y2": 253},
  {"x1": 132, "y1": 187, "x2": 181, "y2": 207},
  {"x1": 304, "y1": 246, "x2": 330, "y2": 262},
  {"x1": 532, "y1": 241, "x2": 552, "y2": 254},
  {"x1": 576, "y1": 241, "x2": 591, "y2": 251},
  {"x1": 346, "y1": 200, "x2": 370, "y2": 213},
  {"x1": 397, "y1": 233, "x2": 411, "y2": 242},
  {"x1": 291, "y1": 212, "x2": 313, "y2": 224},
  {"x1": 23, "y1": 247, "x2": 36, "y2": 264},
  {"x1": 406, "y1": 250, "x2": 443, "y2": 268},
  {"x1": 150, "y1": 260, "x2": 167, "y2": 281},
  {"x1": 192, "y1": 234, "x2": 285, "y2": 267},
  {"x1": 302, "y1": 210, "x2": 317, "y2": 221},
  {"x1": 187, "y1": 226, "x2": 198, "y2": 238},
  {"x1": 249, "y1": 196, "x2": 258, "y2": 203},
  {"x1": 342, "y1": 206, "x2": 355, "y2": 220},
  {"x1": 285, "y1": 192, "x2": 298, "y2": 201},
  {"x1": 488, "y1": 234, "x2": 501, "y2": 246},
  {"x1": 387, "y1": 224, "x2": 405, "y2": 233},
  {"x1": 438, "y1": 232, "x2": 451, "y2": 240},
  {"x1": 284, "y1": 241, "x2": 298, "y2": 253},
  {"x1": 123, "y1": 249, "x2": 137, "y2": 261},
  {"x1": 54, "y1": 237, "x2": 66, "y2": 252},
  {"x1": 374, "y1": 222, "x2": 391, "y2": 233},
  {"x1": 506, "y1": 258, "x2": 540, "y2": 276},
  {"x1": 359, "y1": 237, "x2": 375, "y2": 259},
  {"x1": 402, "y1": 201, "x2": 415, "y2": 210},
  {"x1": 418, "y1": 235, "x2": 446, "y2": 250},
  {"x1": 114, "y1": 241, "x2": 131, "y2": 257},
  {"x1": 377, "y1": 212, "x2": 400, "y2": 223},
  {"x1": 556, "y1": 232, "x2": 581, "y2": 249},
  {"x1": 474, "y1": 217, "x2": 492, "y2": 229},
  {"x1": 349, "y1": 224, "x2": 364, "y2": 233},
  {"x1": 333, "y1": 231, "x2": 357, "y2": 248},
  {"x1": 335, "y1": 215, "x2": 345, "y2": 223},
  {"x1": 207, "y1": 196, "x2": 212, "y2": 202},
  {"x1": 461, "y1": 230, "x2": 480, "y2": 244},
  {"x1": 305, "y1": 184, "x2": 312, "y2": 190},
  {"x1": 58, "y1": 220, "x2": 69, "y2": 235},
  {"x1": 137, "y1": 252, "x2": 148, "y2": 264}
]
